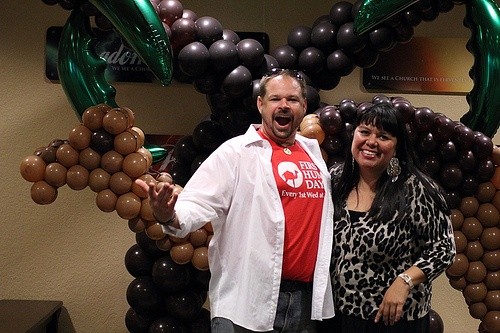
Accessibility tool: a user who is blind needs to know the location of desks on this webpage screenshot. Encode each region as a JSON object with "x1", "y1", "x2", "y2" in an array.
[{"x1": 0, "y1": 299, "x2": 63, "y2": 333}]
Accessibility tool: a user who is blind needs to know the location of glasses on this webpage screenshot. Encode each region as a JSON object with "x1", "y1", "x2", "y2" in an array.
[{"x1": 264, "y1": 68, "x2": 304, "y2": 83}]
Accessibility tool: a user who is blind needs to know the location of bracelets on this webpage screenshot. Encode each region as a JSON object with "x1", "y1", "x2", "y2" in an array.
[{"x1": 151, "y1": 212, "x2": 176, "y2": 226}]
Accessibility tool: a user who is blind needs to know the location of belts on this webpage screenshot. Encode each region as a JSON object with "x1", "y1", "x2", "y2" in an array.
[{"x1": 280, "y1": 280, "x2": 313, "y2": 292}]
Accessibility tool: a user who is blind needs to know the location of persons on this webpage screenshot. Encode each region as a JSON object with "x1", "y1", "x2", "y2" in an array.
[
  {"x1": 328, "y1": 103, "x2": 456, "y2": 333},
  {"x1": 148, "y1": 69, "x2": 333, "y2": 333}
]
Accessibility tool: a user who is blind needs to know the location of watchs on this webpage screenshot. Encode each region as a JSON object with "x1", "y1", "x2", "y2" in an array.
[{"x1": 399, "y1": 273, "x2": 414, "y2": 290}]
